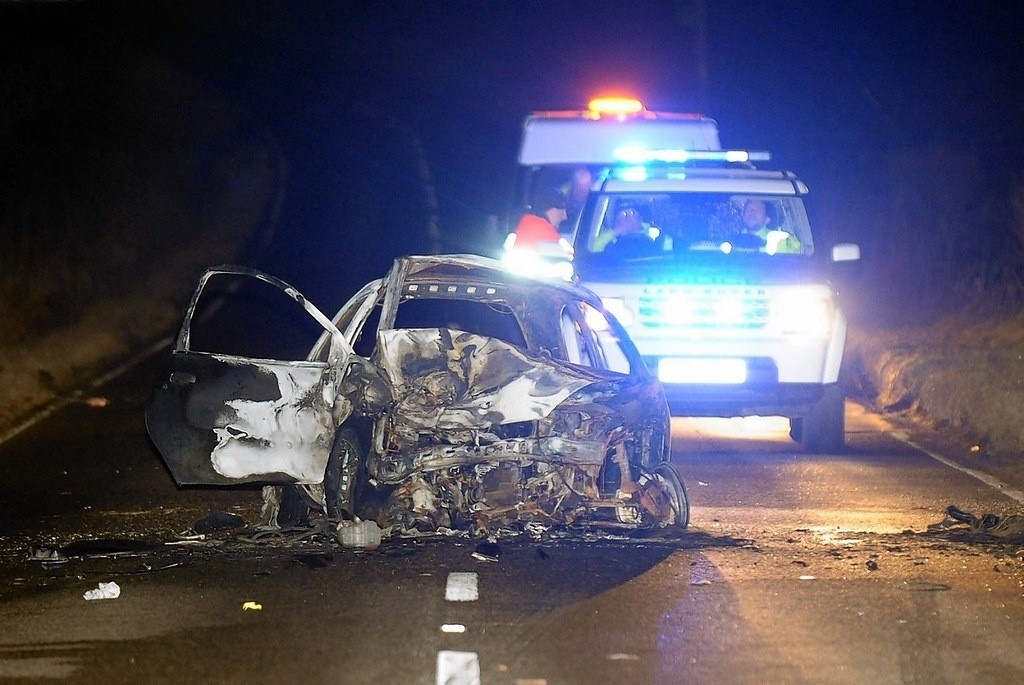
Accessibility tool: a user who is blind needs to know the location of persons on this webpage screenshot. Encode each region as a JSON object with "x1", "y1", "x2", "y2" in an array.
[
  {"x1": 542, "y1": 167, "x2": 592, "y2": 233},
  {"x1": 589, "y1": 199, "x2": 655, "y2": 253},
  {"x1": 739, "y1": 199, "x2": 802, "y2": 254}
]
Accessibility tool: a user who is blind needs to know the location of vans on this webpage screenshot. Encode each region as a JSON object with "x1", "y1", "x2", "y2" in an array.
[
  {"x1": 510, "y1": 109, "x2": 725, "y2": 242},
  {"x1": 571, "y1": 147, "x2": 862, "y2": 453}
]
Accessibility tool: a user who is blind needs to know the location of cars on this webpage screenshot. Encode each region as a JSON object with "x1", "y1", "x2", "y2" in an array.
[{"x1": 143, "y1": 251, "x2": 687, "y2": 538}]
